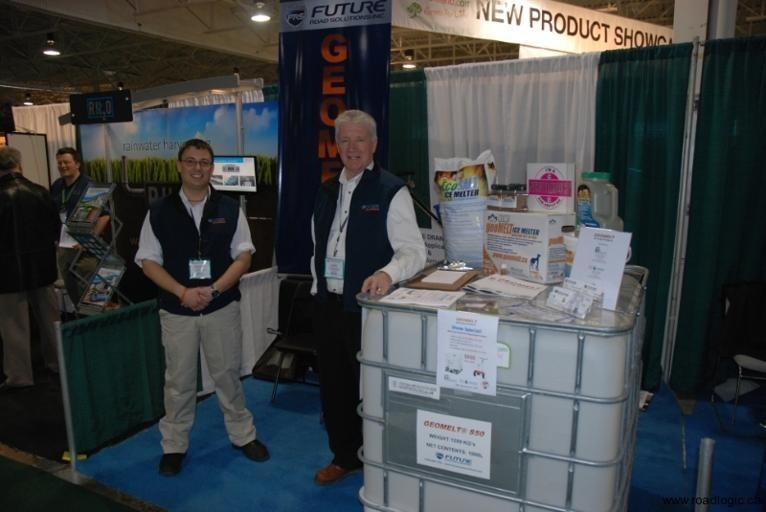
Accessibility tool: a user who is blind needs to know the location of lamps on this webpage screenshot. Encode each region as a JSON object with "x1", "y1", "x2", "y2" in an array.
[
  {"x1": 247, "y1": 1, "x2": 274, "y2": 24},
  {"x1": 398, "y1": 53, "x2": 419, "y2": 72},
  {"x1": 36, "y1": 30, "x2": 64, "y2": 58}
]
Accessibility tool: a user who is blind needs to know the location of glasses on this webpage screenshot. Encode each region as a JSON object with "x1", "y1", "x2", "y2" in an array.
[{"x1": 180, "y1": 157, "x2": 211, "y2": 167}]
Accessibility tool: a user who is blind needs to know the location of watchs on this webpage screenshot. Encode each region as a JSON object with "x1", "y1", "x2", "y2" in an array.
[{"x1": 209, "y1": 283, "x2": 221, "y2": 299}]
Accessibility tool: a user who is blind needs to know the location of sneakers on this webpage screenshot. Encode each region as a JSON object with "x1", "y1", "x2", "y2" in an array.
[
  {"x1": 159, "y1": 453, "x2": 187, "y2": 477},
  {"x1": 37, "y1": 368, "x2": 59, "y2": 379},
  {"x1": 232, "y1": 440, "x2": 270, "y2": 461},
  {"x1": 0, "y1": 381, "x2": 36, "y2": 391}
]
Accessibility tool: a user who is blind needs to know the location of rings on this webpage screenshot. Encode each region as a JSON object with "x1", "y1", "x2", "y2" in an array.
[{"x1": 377, "y1": 287, "x2": 382, "y2": 291}]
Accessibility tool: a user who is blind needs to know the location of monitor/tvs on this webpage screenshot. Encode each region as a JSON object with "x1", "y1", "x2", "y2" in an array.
[{"x1": 212, "y1": 153, "x2": 258, "y2": 196}]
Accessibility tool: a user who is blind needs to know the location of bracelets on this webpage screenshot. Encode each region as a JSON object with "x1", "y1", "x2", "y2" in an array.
[{"x1": 180, "y1": 287, "x2": 188, "y2": 302}]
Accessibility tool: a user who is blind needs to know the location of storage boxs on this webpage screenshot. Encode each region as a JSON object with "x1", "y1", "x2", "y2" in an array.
[{"x1": 477, "y1": 201, "x2": 581, "y2": 285}]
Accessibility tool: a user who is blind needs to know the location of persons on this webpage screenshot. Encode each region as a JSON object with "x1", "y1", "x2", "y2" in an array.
[
  {"x1": 0, "y1": 145, "x2": 62, "y2": 394},
  {"x1": 49, "y1": 147, "x2": 97, "y2": 315},
  {"x1": 134, "y1": 139, "x2": 270, "y2": 477},
  {"x1": 307, "y1": 108, "x2": 427, "y2": 486}
]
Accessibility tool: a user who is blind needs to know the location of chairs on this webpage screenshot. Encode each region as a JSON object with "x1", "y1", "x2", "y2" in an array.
[
  {"x1": 704, "y1": 279, "x2": 766, "y2": 434},
  {"x1": 265, "y1": 277, "x2": 325, "y2": 425}
]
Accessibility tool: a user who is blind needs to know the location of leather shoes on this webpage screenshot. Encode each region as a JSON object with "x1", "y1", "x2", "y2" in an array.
[{"x1": 314, "y1": 461, "x2": 363, "y2": 485}]
